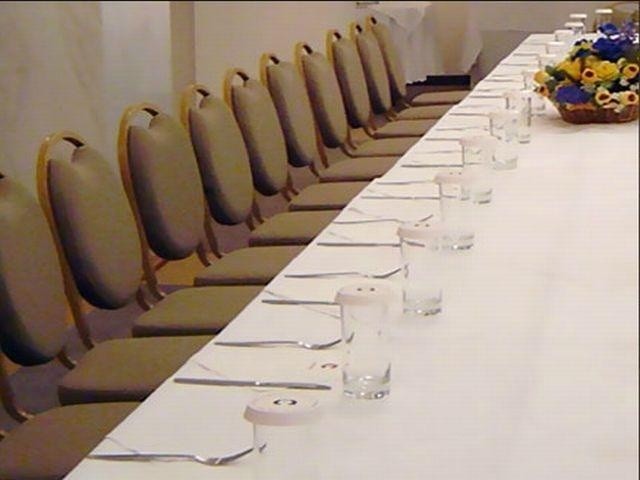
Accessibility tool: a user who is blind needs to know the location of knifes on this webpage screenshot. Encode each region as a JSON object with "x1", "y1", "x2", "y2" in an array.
[
  {"x1": 315, "y1": 239, "x2": 406, "y2": 246},
  {"x1": 173, "y1": 377, "x2": 331, "y2": 392},
  {"x1": 258, "y1": 297, "x2": 345, "y2": 308}
]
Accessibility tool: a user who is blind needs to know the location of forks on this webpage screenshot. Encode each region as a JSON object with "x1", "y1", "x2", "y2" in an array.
[
  {"x1": 333, "y1": 213, "x2": 434, "y2": 227},
  {"x1": 87, "y1": 447, "x2": 253, "y2": 466},
  {"x1": 214, "y1": 337, "x2": 342, "y2": 351},
  {"x1": 285, "y1": 263, "x2": 402, "y2": 279}
]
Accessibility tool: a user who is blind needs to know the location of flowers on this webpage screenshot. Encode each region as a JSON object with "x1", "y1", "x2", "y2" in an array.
[{"x1": 532, "y1": 13, "x2": 639, "y2": 110}]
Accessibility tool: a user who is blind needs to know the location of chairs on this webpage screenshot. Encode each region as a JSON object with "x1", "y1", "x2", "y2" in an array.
[{"x1": 0, "y1": 10, "x2": 472, "y2": 480}]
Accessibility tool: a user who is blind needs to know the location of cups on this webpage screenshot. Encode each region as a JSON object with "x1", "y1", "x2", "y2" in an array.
[
  {"x1": 490, "y1": 92, "x2": 531, "y2": 172},
  {"x1": 243, "y1": 394, "x2": 326, "y2": 480},
  {"x1": 396, "y1": 221, "x2": 449, "y2": 316},
  {"x1": 565, "y1": 9, "x2": 613, "y2": 37},
  {"x1": 333, "y1": 283, "x2": 393, "y2": 400},
  {"x1": 522, "y1": 30, "x2": 575, "y2": 116},
  {"x1": 437, "y1": 134, "x2": 495, "y2": 250}
]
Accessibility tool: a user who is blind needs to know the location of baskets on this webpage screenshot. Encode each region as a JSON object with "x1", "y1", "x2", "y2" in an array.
[{"x1": 551, "y1": 95, "x2": 640, "y2": 126}]
